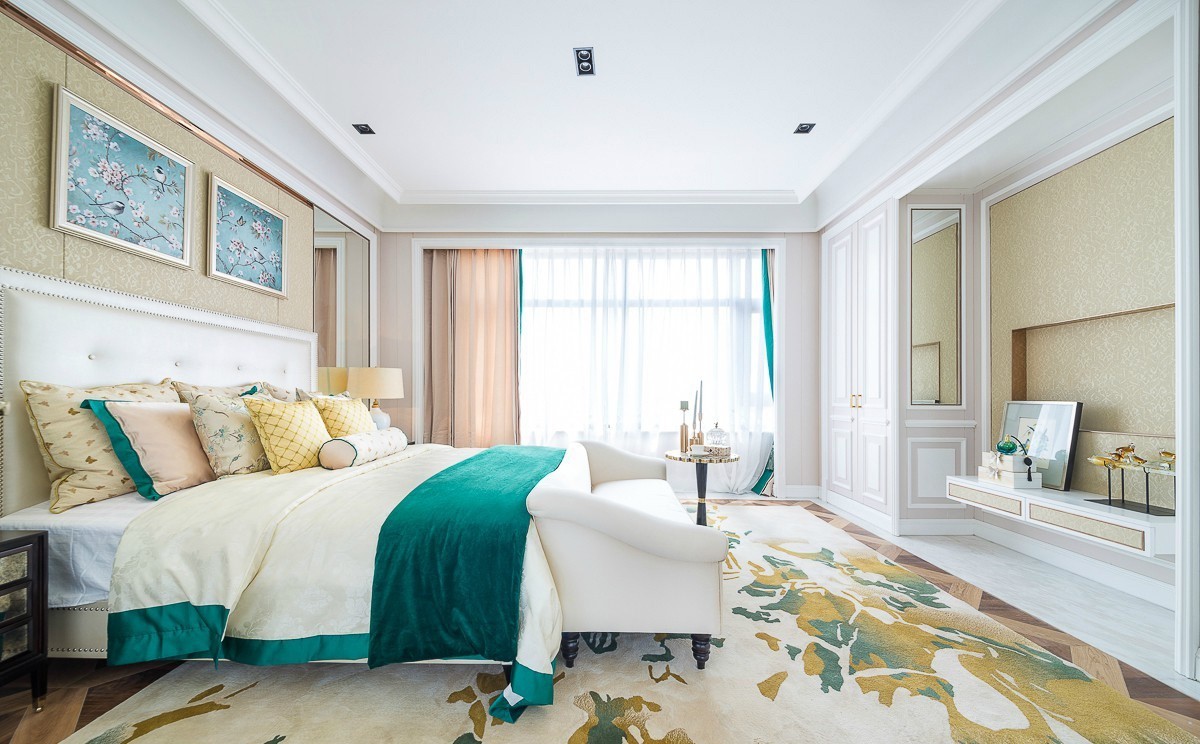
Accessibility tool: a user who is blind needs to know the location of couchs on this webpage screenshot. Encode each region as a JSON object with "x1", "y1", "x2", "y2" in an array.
[{"x1": 525, "y1": 438, "x2": 732, "y2": 671}]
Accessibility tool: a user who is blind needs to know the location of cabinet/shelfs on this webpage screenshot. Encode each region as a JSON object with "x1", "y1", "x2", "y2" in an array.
[{"x1": 820, "y1": 194, "x2": 901, "y2": 534}]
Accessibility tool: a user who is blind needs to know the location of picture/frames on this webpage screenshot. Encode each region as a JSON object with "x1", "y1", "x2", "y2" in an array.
[
  {"x1": 48, "y1": 83, "x2": 198, "y2": 272},
  {"x1": 205, "y1": 171, "x2": 289, "y2": 301},
  {"x1": 998, "y1": 398, "x2": 1084, "y2": 491}
]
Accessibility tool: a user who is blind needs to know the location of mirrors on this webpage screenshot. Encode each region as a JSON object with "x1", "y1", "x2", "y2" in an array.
[
  {"x1": 310, "y1": 204, "x2": 369, "y2": 398},
  {"x1": 906, "y1": 203, "x2": 966, "y2": 409}
]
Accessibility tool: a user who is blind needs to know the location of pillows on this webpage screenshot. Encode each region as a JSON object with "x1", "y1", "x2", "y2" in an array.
[
  {"x1": 317, "y1": 426, "x2": 408, "y2": 470},
  {"x1": 242, "y1": 397, "x2": 332, "y2": 476},
  {"x1": 296, "y1": 389, "x2": 352, "y2": 403},
  {"x1": 171, "y1": 380, "x2": 263, "y2": 403},
  {"x1": 80, "y1": 398, "x2": 218, "y2": 502},
  {"x1": 311, "y1": 395, "x2": 377, "y2": 439},
  {"x1": 263, "y1": 381, "x2": 323, "y2": 402},
  {"x1": 17, "y1": 377, "x2": 181, "y2": 514},
  {"x1": 180, "y1": 387, "x2": 274, "y2": 481}
]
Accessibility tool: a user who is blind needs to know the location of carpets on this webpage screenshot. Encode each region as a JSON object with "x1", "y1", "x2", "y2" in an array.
[{"x1": 59, "y1": 504, "x2": 1200, "y2": 744}]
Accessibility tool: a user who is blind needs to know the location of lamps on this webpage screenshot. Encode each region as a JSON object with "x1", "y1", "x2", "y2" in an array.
[
  {"x1": 317, "y1": 365, "x2": 348, "y2": 396},
  {"x1": 348, "y1": 365, "x2": 405, "y2": 430}
]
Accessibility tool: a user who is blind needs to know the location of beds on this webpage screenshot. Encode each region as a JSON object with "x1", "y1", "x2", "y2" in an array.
[{"x1": 0, "y1": 265, "x2": 569, "y2": 725}]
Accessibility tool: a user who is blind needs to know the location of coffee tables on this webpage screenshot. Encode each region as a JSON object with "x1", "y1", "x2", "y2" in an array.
[{"x1": 664, "y1": 449, "x2": 739, "y2": 526}]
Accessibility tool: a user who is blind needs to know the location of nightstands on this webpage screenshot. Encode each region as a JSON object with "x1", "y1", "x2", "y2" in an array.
[{"x1": 0, "y1": 528, "x2": 50, "y2": 715}]
